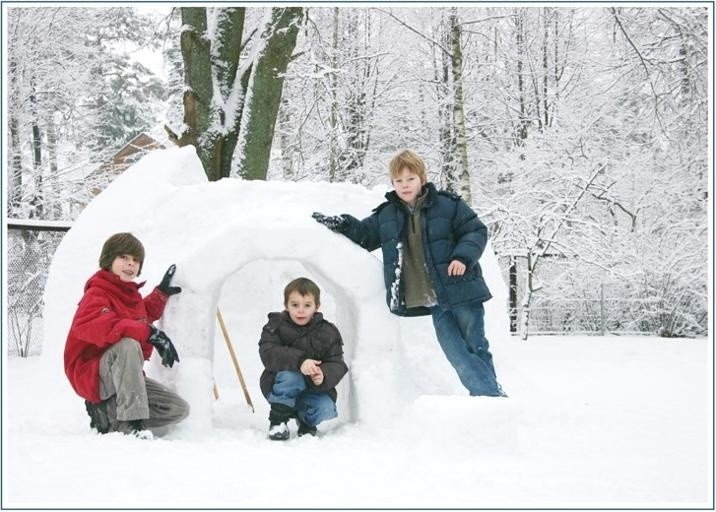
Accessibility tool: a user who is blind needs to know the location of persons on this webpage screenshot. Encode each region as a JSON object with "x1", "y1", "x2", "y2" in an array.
[
  {"x1": 61, "y1": 231, "x2": 190, "y2": 444},
  {"x1": 256, "y1": 276, "x2": 347, "y2": 442},
  {"x1": 308, "y1": 148, "x2": 507, "y2": 400}
]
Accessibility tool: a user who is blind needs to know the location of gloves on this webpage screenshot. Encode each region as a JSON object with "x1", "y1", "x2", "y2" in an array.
[
  {"x1": 157, "y1": 265, "x2": 182, "y2": 296},
  {"x1": 312, "y1": 209, "x2": 352, "y2": 233},
  {"x1": 147, "y1": 322, "x2": 180, "y2": 368}
]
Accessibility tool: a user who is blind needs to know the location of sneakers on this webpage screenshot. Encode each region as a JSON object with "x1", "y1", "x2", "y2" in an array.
[
  {"x1": 267, "y1": 417, "x2": 290, "y2": 441},
  {"x1": 126, "y1": 423, "x2": 151, "y2": 439},
  {"x1": 85, "y1": 400, "x2": 111, "y2": 434},
  {"x1": 297, "y1": 426, "x2": 317, "y2": 439}
]
